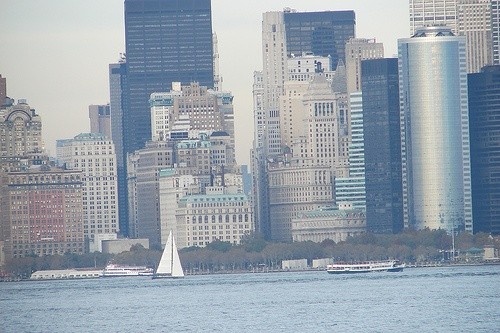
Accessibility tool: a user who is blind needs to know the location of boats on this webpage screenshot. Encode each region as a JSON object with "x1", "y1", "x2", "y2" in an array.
[
  {"x1": 327, "y1": 260, "x2": 404, "y2": 274},
  {"x1": 99, "y1": 265, "x2": 153, "y2": 275}
]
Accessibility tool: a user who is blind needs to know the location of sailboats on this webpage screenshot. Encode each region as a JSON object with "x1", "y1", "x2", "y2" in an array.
[{"x1": 152, "y1": 228, "x2": 185, "y2": 279}]
